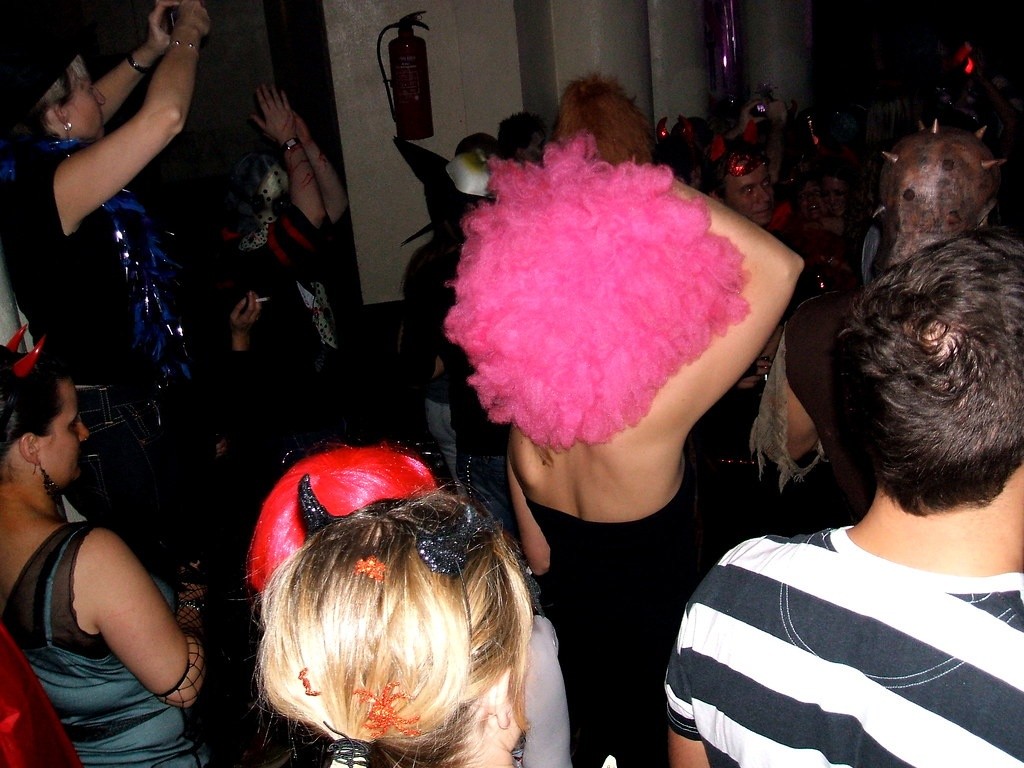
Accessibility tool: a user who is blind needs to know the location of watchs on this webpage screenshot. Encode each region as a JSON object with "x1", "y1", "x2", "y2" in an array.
[
  {"x1": 281, "y1": 136, "x2": 299, "y2": 152},
  {"x1": 173, "y1": 596, "x2": 207, "y2": 613}
]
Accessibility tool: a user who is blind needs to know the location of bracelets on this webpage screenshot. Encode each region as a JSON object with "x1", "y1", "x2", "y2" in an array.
[
  {"x1": 165, "y1": 38, "x2": 201, "y2": 58},
  {"x1": 126, "y1": 52, "x2": 153, "y2": 73}
]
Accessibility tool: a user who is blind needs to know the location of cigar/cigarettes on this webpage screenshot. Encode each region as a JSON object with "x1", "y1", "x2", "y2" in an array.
[{"x1": 253, "y1": 296, "x2": 271, "y2": 304}]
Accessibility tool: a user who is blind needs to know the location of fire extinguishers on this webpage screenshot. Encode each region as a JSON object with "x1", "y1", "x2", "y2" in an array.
[{"x1": 376, "y1": 8, "x2": 434, "y2": 140}]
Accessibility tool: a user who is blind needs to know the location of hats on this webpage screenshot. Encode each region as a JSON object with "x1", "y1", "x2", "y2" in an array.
[{"x1": 0, "y1": 18, "x2": 100, "y2": 139}]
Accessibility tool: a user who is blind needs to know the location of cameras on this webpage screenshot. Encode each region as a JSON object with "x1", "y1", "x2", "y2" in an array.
[{"x1": 166, "y1": 7, "x2": 179, "y2": 35}]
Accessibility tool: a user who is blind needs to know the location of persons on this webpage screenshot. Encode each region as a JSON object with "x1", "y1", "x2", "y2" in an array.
[
  {"x1": 0, "y1": 1, "x2": 249, "y2": 750},
  {"x1": 251, "y1": 473, "x2": 554, "y2": 768},
  {"x1": 152, "y1": 66, "x2": 1024, "y2": 768},
  {"x1": 0, "y1": 342, "x2": 217, "y2": 768}
]
